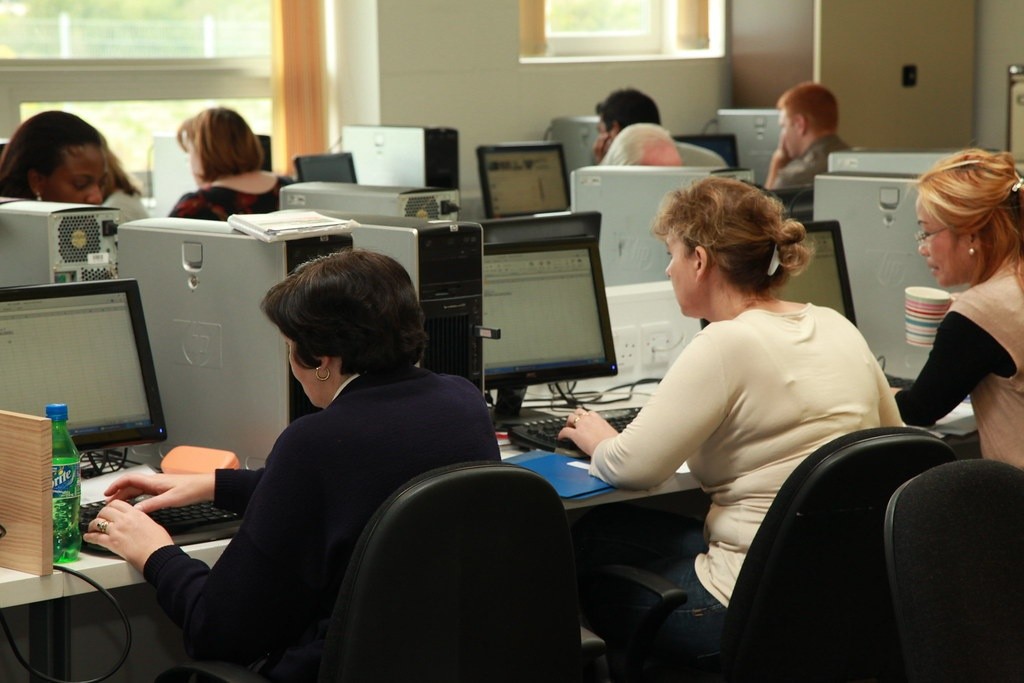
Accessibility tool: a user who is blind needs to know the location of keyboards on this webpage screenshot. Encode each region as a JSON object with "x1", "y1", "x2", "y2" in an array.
[
  {"x1": 73, "y1": 496, "x2": 244, "y2": 556},
  {"x1": 507, "y1": 405, "x2": 645, "y2": 458}
]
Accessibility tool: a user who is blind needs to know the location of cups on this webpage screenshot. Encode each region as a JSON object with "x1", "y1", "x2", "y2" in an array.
[{"x1": 905, "y1": 286, "x2": 953, "y2": 348}]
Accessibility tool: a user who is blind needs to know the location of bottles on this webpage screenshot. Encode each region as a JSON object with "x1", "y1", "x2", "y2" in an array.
[{"x1": 45, "y1": 403, "x2": 81, "y2": 564}]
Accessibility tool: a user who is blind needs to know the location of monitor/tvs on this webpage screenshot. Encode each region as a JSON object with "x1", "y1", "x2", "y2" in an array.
[
  {"x1": 672, "y1": 133, "x2": 739, "y2": 168},
  {"x1": 484, "y1": 234, "x2": 618, "y2": 430},
  {"x1": 293, "y1": 153, "x2": 356, "y2": 184},
  {"x1": 476, "y1": 142, "x2": 571, "y2": 220},
  {"x1": 479, "y1": 210, "x2": 603, "y2": 242},
  {"x1": 0, "y1": 277, "x2": 170, "y2": 449},
  {"x1": 700, "y1": 218, "x2": 856, "y2": 331}
]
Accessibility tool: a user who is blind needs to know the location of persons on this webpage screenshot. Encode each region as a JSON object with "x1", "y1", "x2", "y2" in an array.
[
  {"x1": 1, "y1": 111, "x2": 148, "y2": 225},
  {"x1": 594, "y1": 91, "x2": 725, "y2": 166},
  {"x1": 83, "y1": 253, "x2": 502, "y2": 682},
  {"x1": 556, "y1": 177, "x2": 906, "y2": 675},
  {"x1": 164, "y1": 108, "x2": 294, "y2": 221},
  {"x1": 891, "y1": 149, "x2": 1024, "y2": 470},
  {"x1": 764, "y1": 82, "x2": 848, "y2": 188}
]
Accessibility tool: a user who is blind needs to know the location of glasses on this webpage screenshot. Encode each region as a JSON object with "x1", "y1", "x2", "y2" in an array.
[{"x1": 915, "y1": 225, "x2": 949, "y2": 242}]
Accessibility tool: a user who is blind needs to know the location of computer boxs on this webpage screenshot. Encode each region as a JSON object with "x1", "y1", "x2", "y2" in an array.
[{"x1": 0, "y1": 108, "x2": 986, "y2": 474}]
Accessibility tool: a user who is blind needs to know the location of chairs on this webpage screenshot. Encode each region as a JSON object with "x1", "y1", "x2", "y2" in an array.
[
  {"x1": 881, "y1": 458, "x2": 1024, "y2": 683},
  {"x1": 151, "y1": 461, "x2": 610, "y2": 683},
  {"x1": 595, "y1": 426, "x2": 963, "y2": 683}
]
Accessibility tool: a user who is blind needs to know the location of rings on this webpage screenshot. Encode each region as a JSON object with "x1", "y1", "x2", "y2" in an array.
[
  {"x1": 97, "y1": 521, "x2": 110, "y2": 533},
  {"x1": 574, "y1": 417, "x2": 579, "y2": 426},
  {"x1": 583, "y1": 412, "x2": 589, "y2": 415}
]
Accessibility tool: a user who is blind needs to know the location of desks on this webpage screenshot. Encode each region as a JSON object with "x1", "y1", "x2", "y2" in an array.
[{"x1": 0, "y1": 376, "x2": 981, "y2": 683}]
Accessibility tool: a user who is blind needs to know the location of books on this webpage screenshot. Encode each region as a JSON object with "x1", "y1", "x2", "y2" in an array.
[{"x1": 228, "y1": 211, "x2": 358, "y2": 243}]
